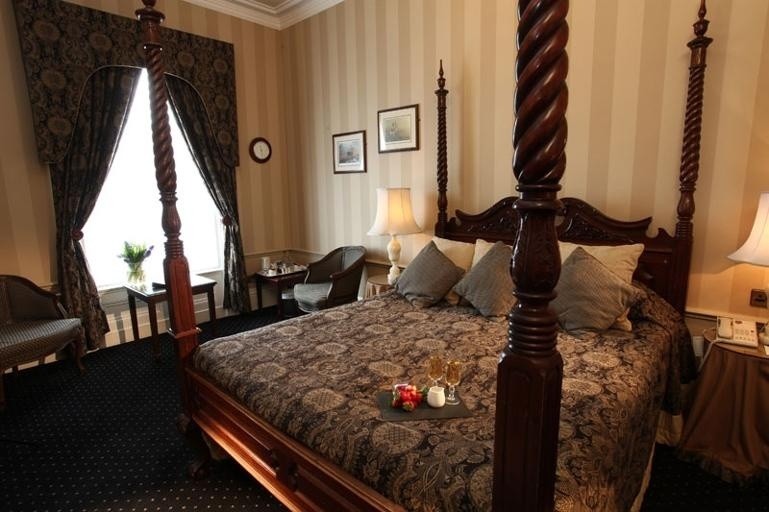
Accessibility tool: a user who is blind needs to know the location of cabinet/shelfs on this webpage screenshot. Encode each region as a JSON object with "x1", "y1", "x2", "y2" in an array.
[
  {"x1": 276, "y1": 260, "x2": 296, "y2": 275},
  {"x1": 427, "y1": 386, "x2": 446, "y2": 408}
]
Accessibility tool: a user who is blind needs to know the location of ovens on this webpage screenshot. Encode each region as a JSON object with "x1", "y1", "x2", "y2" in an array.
[{"x1": 131, "y1": 0, "x2": 714, "y2": 511}]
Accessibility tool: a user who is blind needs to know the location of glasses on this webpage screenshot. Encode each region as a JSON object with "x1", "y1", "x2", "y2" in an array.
[
  {"x1": 366, "y1": 186, "x2": 424, "y2": 287},
  {"x1": 726, "y1": 191, "x2": 769, "y2": 269}
]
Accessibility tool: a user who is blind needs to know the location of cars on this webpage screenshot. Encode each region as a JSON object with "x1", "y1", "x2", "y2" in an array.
[{"x1": 261, "y1": 257, "x2": 271, "y2": 271}]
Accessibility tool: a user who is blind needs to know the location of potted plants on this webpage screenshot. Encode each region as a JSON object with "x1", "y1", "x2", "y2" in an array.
[
  {"x1": 376, "y1": 103, "x2": 421, "y2": 154},
  {"x1": 330, "y1": 130, "x2": 367, "y2": 175}
]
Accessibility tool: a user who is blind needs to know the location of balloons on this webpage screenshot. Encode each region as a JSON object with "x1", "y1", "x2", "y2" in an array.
[{"x1": 247, "y1": 135, "x2": 273, "y2": 165}]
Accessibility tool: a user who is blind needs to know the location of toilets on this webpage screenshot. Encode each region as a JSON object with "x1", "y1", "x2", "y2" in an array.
[{"x1": 717, "y1": 315, "x2": 759, "y2": 347}]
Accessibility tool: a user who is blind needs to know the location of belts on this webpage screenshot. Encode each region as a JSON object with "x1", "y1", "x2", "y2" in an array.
[
  {"x1": 442, "y1": 360, "x2": 463, "y2": 406},
  {"x1": 426, "y1": 353, "x2": 444, "y2": 387}
]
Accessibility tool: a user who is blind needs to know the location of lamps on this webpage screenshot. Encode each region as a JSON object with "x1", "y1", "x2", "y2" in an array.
[
  {"x1": 291, "y1": 244, "x2": 367, "y2": 314},
  {"x1": 0, "y1": 273, "x2": 85, "y2": 405}
]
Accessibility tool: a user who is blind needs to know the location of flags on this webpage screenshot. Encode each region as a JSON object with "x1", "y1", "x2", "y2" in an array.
[{"x1": 125, "y1": 261, "x2": 150, "y2": 291}]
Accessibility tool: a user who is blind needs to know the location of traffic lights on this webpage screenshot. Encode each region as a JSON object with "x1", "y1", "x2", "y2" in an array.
[
  {"x1": 458, "y1": 238, "x2": 512, "y2": 307},
  {"x1": 390, "y1": 238, "x2": 466, "y2": 311},
  {"x1": 554, "y1": 239, "x2": 645, "y2": 334},
  {"x1": 632, "y1": 278, "x2": 698, "y2": 395},
  {"x1": 451, "y1": 240, "x2": 521, "y2": 322},
  {"x1": 547, "y1": 244, "x2": 647, "y2": 342},
  {"x1": 430, "y1": 235, "x2": 475, "y2": 308}
]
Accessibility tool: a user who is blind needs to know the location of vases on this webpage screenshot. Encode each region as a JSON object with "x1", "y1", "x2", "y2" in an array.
[{"x1": 117, "y1": 240, "x2": 156, "y2": 284}]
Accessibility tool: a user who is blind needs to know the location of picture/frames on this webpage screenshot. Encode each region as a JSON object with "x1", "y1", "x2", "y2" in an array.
[
  {"x1": 122, "y1": 271, "x2": 222, "y2": 355},
  {"x1": 366, "y1": 274, "x2": 394, "y2": 297},
  {"x1": 674, "y1": 323, "x2": 769, "y2": 490},
  {"x1": 254, "y1": 263, "x2": 311, "y2": 321}
]
